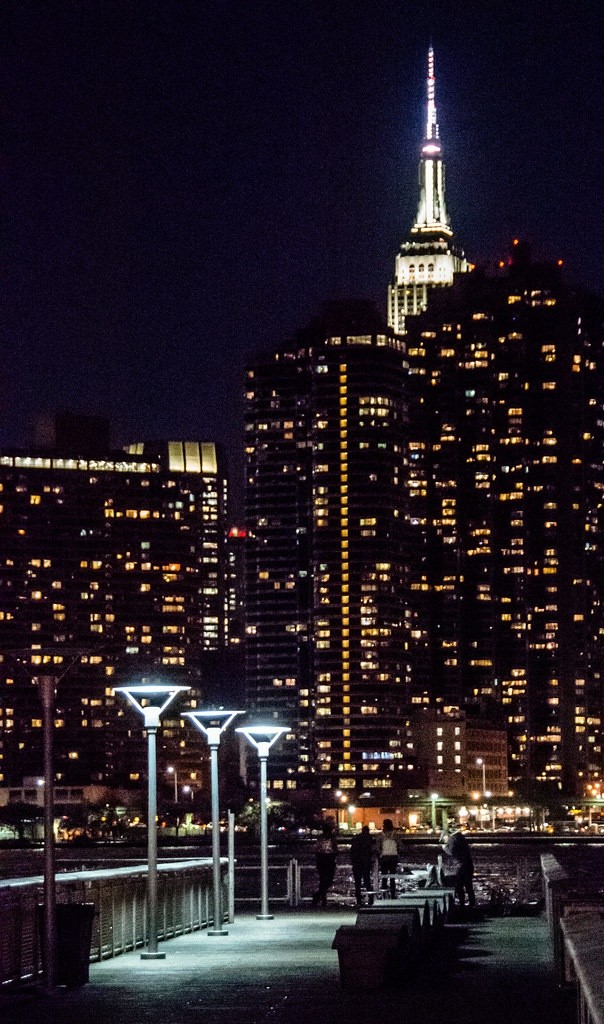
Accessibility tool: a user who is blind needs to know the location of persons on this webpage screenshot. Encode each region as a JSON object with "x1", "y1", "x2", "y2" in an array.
[
  {"x1": 350, "y1": 826, "x2": 377, "y2": 907},
  {"x1": 441, "y1": 829, "x2": 477, "y2": 910},
  {"x1": 313, "y1": 824, "x2": 338, "y2": 906},
  {"x1": 376, "y1": 819, "x2": 402, "y2": 898}
]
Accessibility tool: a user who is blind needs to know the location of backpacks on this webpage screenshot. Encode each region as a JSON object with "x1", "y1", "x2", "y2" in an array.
[{"x1": 381, "y1": 829, "x2": 398, "y2": 856}]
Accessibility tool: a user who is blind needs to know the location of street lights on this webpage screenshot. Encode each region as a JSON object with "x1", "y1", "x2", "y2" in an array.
[
  {"x1": 235, "y1": 725, "x2": 292, "y2": 920},
  {"x1": 432, "y1": 793, "x2": 439, "y2": 833},
  {"x1": 111, "y1": 685, "x2": 193, "y2": 960},
  {"x1": 0, "y1": 648, "x2": 93, "y2": 995},
  {"x1": 179, "y1": 710, "x2": 247, "y2": 936},
  {"x1": 478, "y1": 759, "x2": 486, "y2": 795},
  {"x1": 167, "y1": 767, "x2": 178, "y2": 807}
]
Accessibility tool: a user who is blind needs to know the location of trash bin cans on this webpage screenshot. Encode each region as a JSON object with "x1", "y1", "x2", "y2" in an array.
[{"x1": 35, "y1": 901, "x2": 94, "y2": 988}]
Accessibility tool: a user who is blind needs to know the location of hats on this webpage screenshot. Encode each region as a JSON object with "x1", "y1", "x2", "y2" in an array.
[{"x1": 439, "y1": 828, "x2": 449, "y2": 842}]
